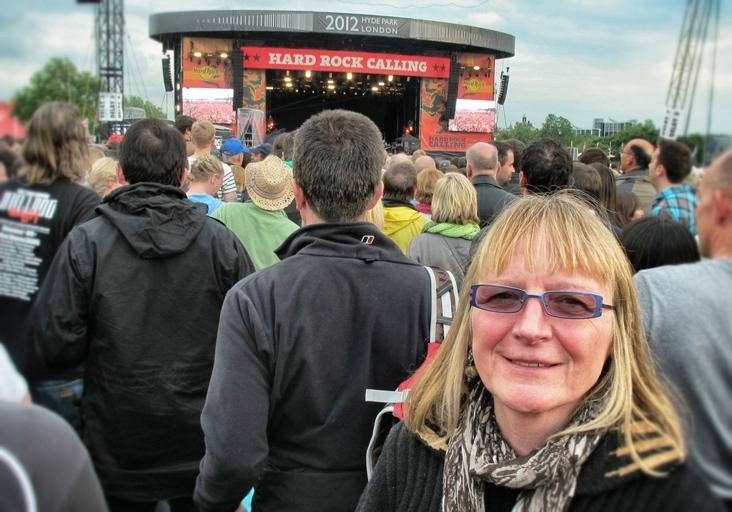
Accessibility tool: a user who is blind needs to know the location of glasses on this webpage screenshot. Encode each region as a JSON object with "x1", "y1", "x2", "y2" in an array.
[{"x1": 469, "y1": 285, "x2": 617, "y2": 320}]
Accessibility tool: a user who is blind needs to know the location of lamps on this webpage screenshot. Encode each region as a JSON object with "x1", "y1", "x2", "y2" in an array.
[
  {"x1": 185, "y1": 50, "x2": 233, "y2": 66},
  {"x1": 266, "y1": 69, "x2": 412, "y2": 98},
  {"x1": 453, "y1": 65, "x2": 491, "y2": 77}
]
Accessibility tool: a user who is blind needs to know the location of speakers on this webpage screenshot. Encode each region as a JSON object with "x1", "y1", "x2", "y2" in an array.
[
  {"x1": 231, "y1": 50, "x2": 243, "y2": 111},
  {"x1": 162, "y1": 59, "x2": 173, "y2": 92},
  {"x1": 498, "y1": 75, "x2": 509, "y2": 105},
  {"x1": 446, "y1": 63, "x2": 460, "y2": 119}
]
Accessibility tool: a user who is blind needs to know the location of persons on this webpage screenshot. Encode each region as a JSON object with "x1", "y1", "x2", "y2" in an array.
[
  {"x1": 175, "y1": 115, "x2": 303, "y2": 268},
  {"x1": 360, "y1": 139, "x2": 530, "y2": 292},
  {"x1": 193, "y1": 108, "x2": 461, "y2": 512},
  {"x1": 357, "y1": 195, "x2": 721, "y2": 512},
  {"x1": 518, "y1": 138, "x2": 701, "y2": 273},
  {"x1": 632, "y1": 150, "x2": 732, "y2": 512},
  {"x1": 25, "y1": 118, "x2": 256, "y2": 511},
  {"x1": 0, "y1": 104, "x2": 122, "y2": 512}
]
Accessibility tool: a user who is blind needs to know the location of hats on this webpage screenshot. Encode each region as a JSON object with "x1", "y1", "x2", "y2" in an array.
[
  {"x1": 223, "y1": 138, "x2": 250, "y2": 155},
  {"x1": 249, "y1": 143, "x2": 272, "y2": 154},
  {"x1": 247, "y1": 157, "x2": 295, "y2": 211}
]
